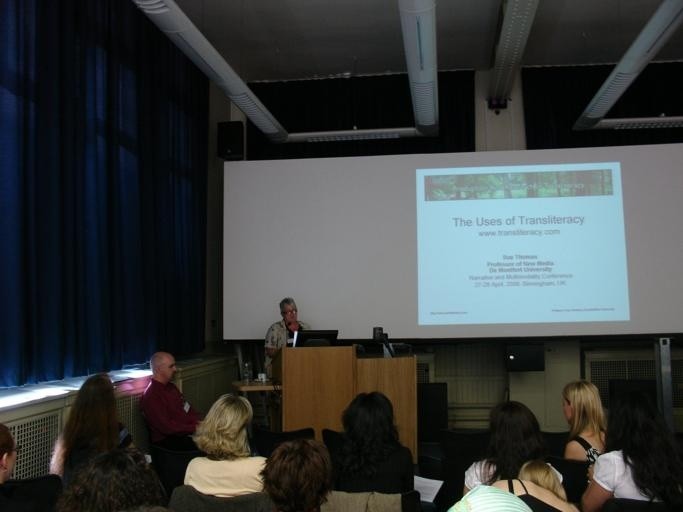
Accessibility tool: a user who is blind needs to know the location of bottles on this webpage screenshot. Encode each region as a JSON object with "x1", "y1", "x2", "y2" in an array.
[{"x1": 243, "y1": 363, "x2": 250, "y2": 385}]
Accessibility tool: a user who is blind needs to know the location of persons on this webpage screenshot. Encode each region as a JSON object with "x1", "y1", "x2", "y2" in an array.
[
  {"x1": 330, "y1": 391, "x2": 414, "y2": 494},
  {"x1": 139, "y1": 352, "x2": 203, "y2": 469},
  {"x1": 264, "y1": 295, "x2": 311, "y2": 379},
  {"x1": 1, "y1": 418, "x2": 64, "y2": 512},
  {"x1": 582, "y1": 362, "x2": 683, "y2": 512},
  {"x1": 184, "y1": 394, "x2": 268, "y2": 497},
  {"x1": 448, "y1": 460, "x2": 579, "y2": 512},
  {"x1": 259, "y1": 441, "x2": 333, "y2": 511},
  {"x1": 563, "y1": 380, "x2": 610, "y2": 461},
  {"x1": 51, "y1": 373, "x2": 146, "y2": 477},
  {"x1": 57, "y1": 451, "x2": 166, "y2": 512},
  {"x1": 463, "y1": 401, "x2": 563, "y2": 493}
]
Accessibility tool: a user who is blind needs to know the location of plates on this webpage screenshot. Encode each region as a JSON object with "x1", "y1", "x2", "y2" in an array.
[{"x1": 254, "y1": 378, "x2": 270, "y2": 382}]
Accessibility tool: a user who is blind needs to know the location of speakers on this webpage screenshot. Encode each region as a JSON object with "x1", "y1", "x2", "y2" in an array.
[
  {"x1": 506, "y1": 342, "x2": 544, "y2": 372},
  {"x1": 217, "y1": 121, "x2": 244, "y2": 161},
  {"x1": 417, "y1": 383, "x2": 448, "y2": 442}
]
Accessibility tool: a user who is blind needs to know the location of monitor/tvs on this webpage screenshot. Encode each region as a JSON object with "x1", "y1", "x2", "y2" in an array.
[{"x1": 295, "y1": 330, "x2": 338, "y2": 347}]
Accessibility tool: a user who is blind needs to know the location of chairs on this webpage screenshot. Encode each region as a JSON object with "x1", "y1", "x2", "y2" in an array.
[
  {"x1": 169, "y1": 485, "x2": 274, "y2": 512},
  {"x1": 320, "y1": 490, "x2": 421, "y2": 512}
]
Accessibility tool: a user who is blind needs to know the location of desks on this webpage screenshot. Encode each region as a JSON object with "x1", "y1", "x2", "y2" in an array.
[{"x1": 232, "y1": 379, "x2": 282, "y2": 438}]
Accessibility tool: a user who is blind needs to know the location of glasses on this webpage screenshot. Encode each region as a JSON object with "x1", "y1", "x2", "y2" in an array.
[
  {"x1": 112, "y1": 385, "x2": 116, "y2": 390},
  {"x1": 12, "y1": 444, "x2": 21, "y2": 453},
  {"x1": 284, "y1": 310, "x2": 296, "y2": 314}
]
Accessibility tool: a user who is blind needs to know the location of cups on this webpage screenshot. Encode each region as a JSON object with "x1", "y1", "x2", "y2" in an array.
[{"x1": 257, "y1": 374, "x2": 266, "y2": 380}]
